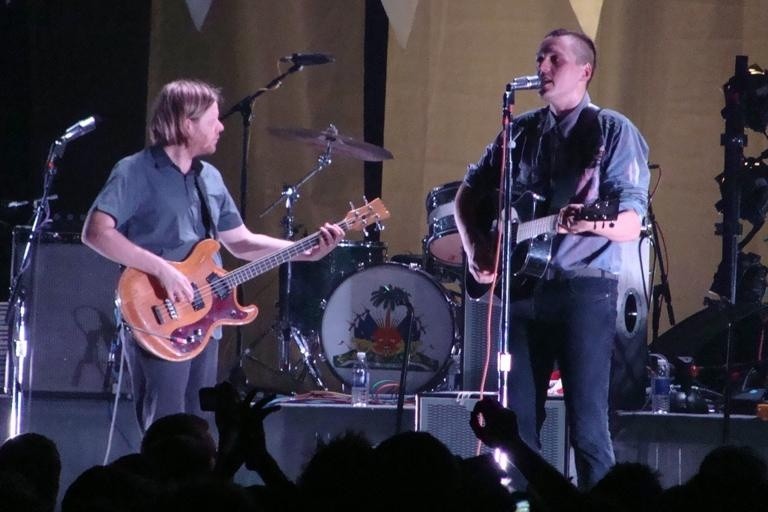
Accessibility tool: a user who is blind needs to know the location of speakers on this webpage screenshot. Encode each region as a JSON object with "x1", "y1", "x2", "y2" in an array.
[
  {"x1": 422, "y1": 391, "x2": 572, "y2": 481},
  {"x1": 8, "y1": 225, "x2": 137, "y2": 397},
  {"x1": 463, "y1": 236, "x2": 657, "y2": 409}
]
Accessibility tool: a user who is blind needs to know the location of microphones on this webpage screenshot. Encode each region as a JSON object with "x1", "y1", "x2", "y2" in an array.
[
  {"x1": 506, "y1": 72, "x2": 545, "y2": 90},
  {"x1": 60, "y1": 113, "x2": 96, "y2": 138},
  {"x1": 287, "y1": 49, "x2": 335, "y2": 66}
]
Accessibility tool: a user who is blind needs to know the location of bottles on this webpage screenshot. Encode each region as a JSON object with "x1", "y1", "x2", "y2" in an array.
[
  {"x1": 650, "y1": 359, "x2": 670, "y2": 414},
  {"x1": 350, "y1": 352, "x2": 370, "y2": 408}
]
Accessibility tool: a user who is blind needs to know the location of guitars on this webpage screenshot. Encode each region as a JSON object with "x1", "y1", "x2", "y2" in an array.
[
  {"x1": 115, "y1": 196, "x2": 389, "y2": 362},
  {"x1": 465, "y1": 192, "x2": 621, "y2": 306}
]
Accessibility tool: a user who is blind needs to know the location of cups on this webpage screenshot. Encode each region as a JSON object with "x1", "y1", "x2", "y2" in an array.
[{"x1": 756, "y1": 403, "x2": 767, "y2": 421}]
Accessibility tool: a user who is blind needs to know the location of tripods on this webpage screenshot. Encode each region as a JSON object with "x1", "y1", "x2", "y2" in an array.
[{"x1": 241, "y1": 157, "x2": 331, "y2": 391}]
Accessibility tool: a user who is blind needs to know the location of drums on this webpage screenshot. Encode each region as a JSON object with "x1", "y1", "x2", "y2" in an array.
[
  {"x1": 426, "y1": 181, "x2": 468, "y2": 267},
  {"x1": 321, "y1": 262, "x2": 457, "y2": 394},
  {"x1": 278, "y1": 242, "x2": 386, "y2": 338}
]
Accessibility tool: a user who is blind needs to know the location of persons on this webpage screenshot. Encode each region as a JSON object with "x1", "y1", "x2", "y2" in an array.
[
  {"x1": 2, "y1": 381, "x2": 766, "y2": 511},
  {"x1": 81, "y1": 78, "x2": 347, "y2": 434},
  {"x1": 453, "y1": 29, "x2": 650, "y2": 493}
]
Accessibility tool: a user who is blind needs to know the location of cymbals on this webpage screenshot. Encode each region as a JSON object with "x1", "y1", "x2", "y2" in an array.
[{"x1": 267, "y1": 127, "x2": 394, "y2": 162}]
